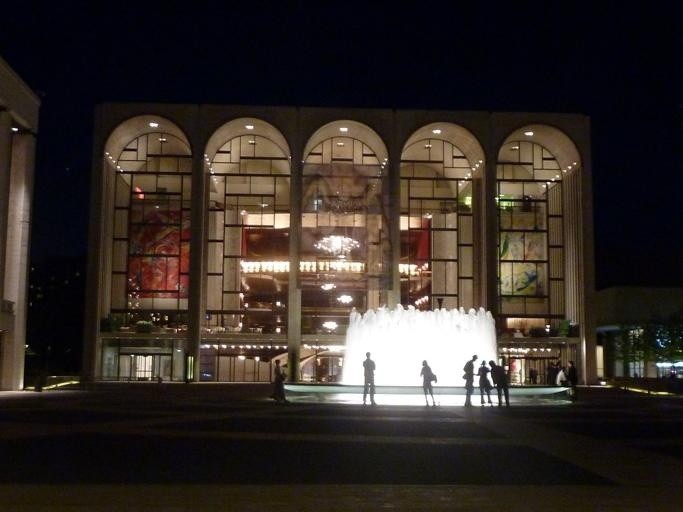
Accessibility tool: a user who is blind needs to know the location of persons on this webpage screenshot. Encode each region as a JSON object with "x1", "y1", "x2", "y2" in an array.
[
  {"x1": 420, "y1": 360, "x2": 437, "y2": 406},
  {"x1": 462, "y1": 354, "x2": 477, "y2": 407},
  {"x1": 362, "y1": 352, "x2": 379, "y2": 407},
  {"x1": 271, "y1": 357, "x2": 290, "y2": 404},
  {"x1": 528, "y1": 358, "x2": 578, "y2": 398},
  {"x1": 488, "y1": 360, "x2": 511, "y2": 408},
  {"x1": 476, "y1": 359, "x2": 492, "y2": 404}
]
